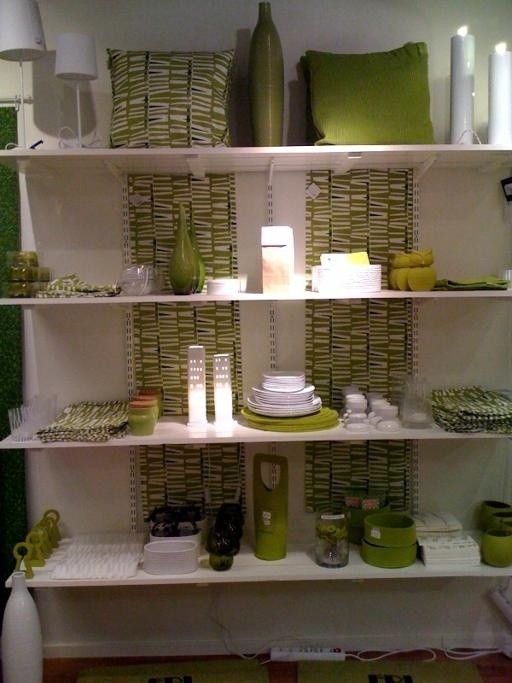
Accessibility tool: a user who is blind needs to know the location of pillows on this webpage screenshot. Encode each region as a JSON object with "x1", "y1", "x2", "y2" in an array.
[
  {"x1": 297, "y1": 38, "x2": 435, "y2": 144},
  {"x1": 103, "y1": 45, "x2": 237, "y2": 148}
]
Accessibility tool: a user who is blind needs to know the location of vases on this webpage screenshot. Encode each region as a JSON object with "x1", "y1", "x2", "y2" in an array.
[
  {"x1": 0, "y1": 569, "x2": 46, "y2": 683},
  {"x1": 245, "y1": 0, "x2": 285, "y2": 146}
]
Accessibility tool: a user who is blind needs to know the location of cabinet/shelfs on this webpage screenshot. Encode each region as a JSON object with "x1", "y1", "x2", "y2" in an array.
[{"x1": 0, "y1": 143, "x2": 512, "y2": 661}]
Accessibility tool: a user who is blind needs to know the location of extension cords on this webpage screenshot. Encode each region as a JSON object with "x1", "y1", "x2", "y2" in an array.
[{"x1": 270, "y1": 647, "x2": 347, "y2": 662}]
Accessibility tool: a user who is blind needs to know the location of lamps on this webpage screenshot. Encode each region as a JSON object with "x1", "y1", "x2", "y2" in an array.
[
  {"x1": 448, "y1": 23, "x2": 510, "y2": 144},
  {"x1": 1, "y1": 0, "x2": 98, "y2": 147}
]
